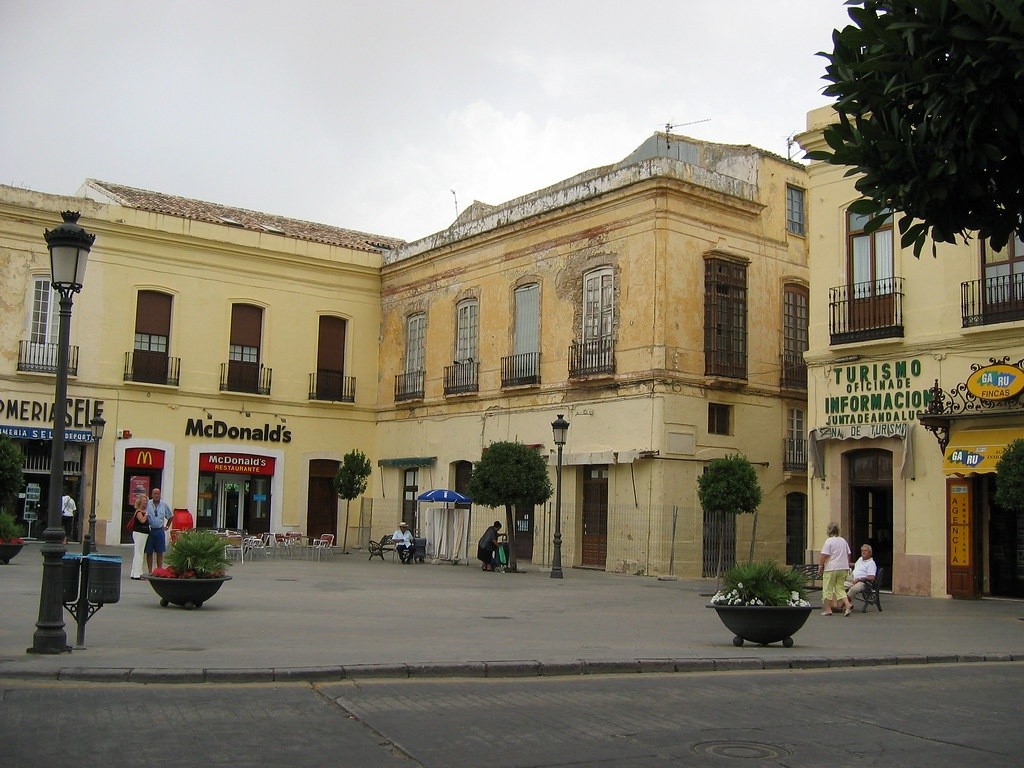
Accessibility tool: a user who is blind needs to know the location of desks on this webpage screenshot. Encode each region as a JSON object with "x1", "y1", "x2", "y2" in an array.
[{"x1": 284, "y1": 536, "x2": 317, "y2": 560}]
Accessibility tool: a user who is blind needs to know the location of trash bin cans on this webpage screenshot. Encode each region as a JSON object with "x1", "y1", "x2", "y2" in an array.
[
  {"x1": 88, "y1": 553, "x2": 123, "y2": 603},
  {"x1": 62, "y1": 552, "x2": 82, "y2": 602}
]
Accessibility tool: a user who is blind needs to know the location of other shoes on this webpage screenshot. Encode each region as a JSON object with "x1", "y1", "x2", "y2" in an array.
[
  {"x1": 407, "y1": 559, "x2": 410, "y2": 564},
  {"x1": 844, "y1": 605, "x2": 853, "y2": 616},
  {"x1": 821, "y1": 612, "x2": 832, "y2": 615},
  {"x1": 831, "y1": 607, "x2": 843, "y2": 612},
  {"x1": 402, "y1": 560, "x2": 405, "y2": 564},
  {"x1": 131, "y1": 577, "x2": 143, "y2": 579}
]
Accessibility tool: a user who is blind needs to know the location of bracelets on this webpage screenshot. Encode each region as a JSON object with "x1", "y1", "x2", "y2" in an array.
[{"x1": 166, "y1": 526, "x2": 168, "y2": 528}]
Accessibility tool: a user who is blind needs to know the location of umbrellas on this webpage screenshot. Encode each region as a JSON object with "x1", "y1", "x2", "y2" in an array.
[{"x1": 417, "y1": 488, "x2": 472, "y2": 559}]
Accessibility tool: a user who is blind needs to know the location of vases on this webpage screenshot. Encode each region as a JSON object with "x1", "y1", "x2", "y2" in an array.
[
  {"x1": 140, "y1": 573, "x2": 232, "y2": 610},
  {"x1": 705, "y1": 602, "x2": 822, "y2": 648},
  {"x1": 0, "y1": 544, "x2": 24, "y2": 565}
]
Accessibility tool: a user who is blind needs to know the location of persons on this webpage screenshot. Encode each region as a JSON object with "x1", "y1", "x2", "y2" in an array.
[
  {"x1": 62, "y1": 486, "x2": 76, "y2": 544},
  {"x1": 820, "y1": 522, "x2": 853, "y2": 616},
  {"x1": 477, "y1": 521, "x2": 505, "y2": 574},
  {"x1": 144, "y1": 488, "x2": 174, "y2": 575},
  {"x1": 392, "y1": 522, "x2": 416, "y2": 564},
  {"x1": 130, "y1": 495, "x2": 150, "y2": 580},
  {"x1": 831, "y1": 544, "x2": 876, "y2": 612}
]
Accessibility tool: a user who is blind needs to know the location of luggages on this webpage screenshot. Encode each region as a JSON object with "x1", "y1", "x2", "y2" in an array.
[{"x1": 494, "y1": 534, "x2": 509, "y2": 571}]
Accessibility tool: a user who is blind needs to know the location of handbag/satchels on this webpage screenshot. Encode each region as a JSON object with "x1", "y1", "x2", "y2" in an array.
[
  {"x1": 500, "y1": 546, "x2": 506, "y2": 564},
  {"x1": 127, "y1": 511, "x2": 142, "y2": 532}
]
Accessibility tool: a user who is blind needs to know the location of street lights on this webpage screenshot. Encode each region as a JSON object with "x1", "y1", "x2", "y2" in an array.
[
  {"x1": 88, "y1": 414, "x2": 107, "y2": 553},
  {"x1": 551, "y1": 413, "x2": 570, "y2": 577},
  {"x1": 22, "y1": 209, "x2": 98, "y2": 656}
]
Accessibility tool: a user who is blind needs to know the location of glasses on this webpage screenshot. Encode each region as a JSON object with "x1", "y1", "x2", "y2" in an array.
[{"x1": 155, "y1": 510, "x2": 157, "y2": 517}]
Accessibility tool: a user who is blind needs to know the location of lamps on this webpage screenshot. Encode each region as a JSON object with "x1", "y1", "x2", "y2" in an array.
[
  {"x1": 240, "y1": 411, "x2": 250, "y2": 417},
  {"x1": 274, "y1": 414, "x2": 286, "y2": 423},
  {"x1": 203, "y1": 408, "x2": 212, "y2": 419}
]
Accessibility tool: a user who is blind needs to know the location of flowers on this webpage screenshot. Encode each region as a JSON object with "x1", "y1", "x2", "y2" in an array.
[
  {"x1": 0, "y1": 507, "x2": 26, "y2": 544},
  {"x1": 149, "y1": 530, "x2": 233, "y2": 579},
  {"x1": 709, "y1": 558, "x2": 814, "y2": 607}
]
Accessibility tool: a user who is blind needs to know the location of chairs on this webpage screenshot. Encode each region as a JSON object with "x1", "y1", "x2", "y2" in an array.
[
  {"x1": 169, "y1": 528, "x2": 335, "y2": 564},
  {"x1": 392, "y1": 542, "x2": 415, "y2": 564}
]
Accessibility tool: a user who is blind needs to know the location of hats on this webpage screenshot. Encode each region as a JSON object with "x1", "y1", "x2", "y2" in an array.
[{"x1": 398, "y1": 522, "x2": 408, "y2": 527}]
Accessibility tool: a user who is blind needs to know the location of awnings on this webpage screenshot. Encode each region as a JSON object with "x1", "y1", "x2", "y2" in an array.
[{"x1": 941, "y1": 423, "x2": 1024, "y2": 478}]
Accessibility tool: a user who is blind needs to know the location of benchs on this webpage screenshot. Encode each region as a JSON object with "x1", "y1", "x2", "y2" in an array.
[
  {"x1": 790, "y1": 565, "x2": 883, "y2": 613},
  {"x1": 368, "y1": 535, "x2": 427, "y2": 564}
]
